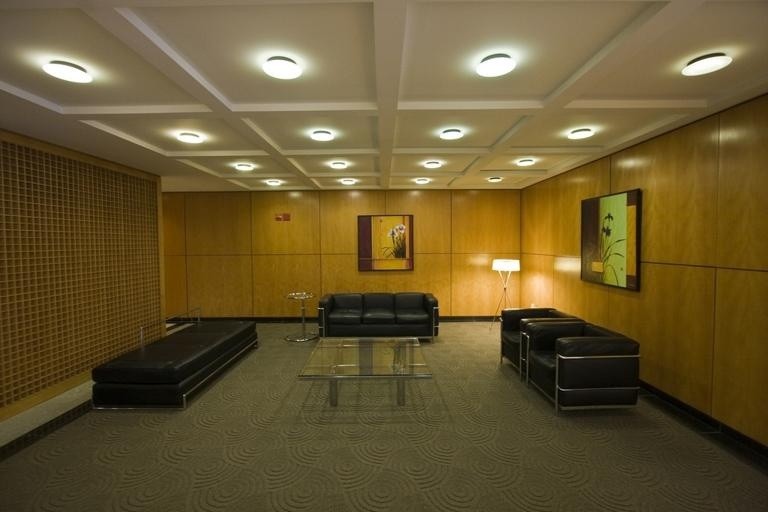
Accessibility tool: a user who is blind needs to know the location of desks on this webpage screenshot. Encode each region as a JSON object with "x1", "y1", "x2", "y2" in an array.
[{"x1": 283, "y1": 291, "x2": 319, "y2": 342}]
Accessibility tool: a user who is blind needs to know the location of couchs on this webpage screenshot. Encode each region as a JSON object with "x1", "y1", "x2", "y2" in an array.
[
  {"x1": 314, "y1": 291, "x2": 441, "y2": 345},
  {"x1": 498, "y1": 305, "x2": 584, "y2": 385},
  {"x1": 525, "y1": 320, "x2": 641, "y2": 419},
  {"x1": 90, "y1": 320, "x2": 260, "y2": 411}
]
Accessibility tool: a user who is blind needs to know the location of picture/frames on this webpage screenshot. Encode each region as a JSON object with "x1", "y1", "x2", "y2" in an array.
[
  {"x1": 580, "y1": 188, "x2": 643, "y2": 293},
  {"x1": 357, "y1": 215, "x2": 415, "y2": 272}
]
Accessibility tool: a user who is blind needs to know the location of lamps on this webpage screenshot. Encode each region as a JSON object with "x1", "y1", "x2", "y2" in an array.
[{"x1": 489, "y1": 259, "x2": 521, "y2": 330}]
